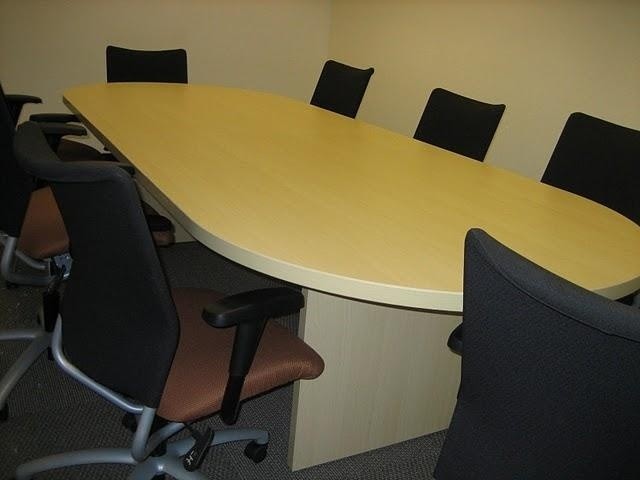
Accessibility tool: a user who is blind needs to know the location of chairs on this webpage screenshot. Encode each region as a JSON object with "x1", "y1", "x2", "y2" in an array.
[
  {"x1": 12, "y1": 119, "x2": 326, "y2": 479},
  {"x1": 1, "y1": 82, "x2": 177, "y2": 406},
  {"x1": 412, "y1": 86, "x2": 507, "y2": 162},
  {"x1": 429, "y1": 225, "x2": 640, "y2": 480},
  {"x1": 540, "y1": 111, "x2": 640, "y2": 227},
  {"x1": 309, "y1": 59, "x2": 375, "y2": 120},
  {"x1": 103, "y1": 46, "x2": 191, "y2": 85}
]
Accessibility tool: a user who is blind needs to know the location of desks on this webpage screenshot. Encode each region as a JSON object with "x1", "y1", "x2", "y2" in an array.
[{"x1": 63, "y1": 84, "x2": 640, "y2": 472}]
